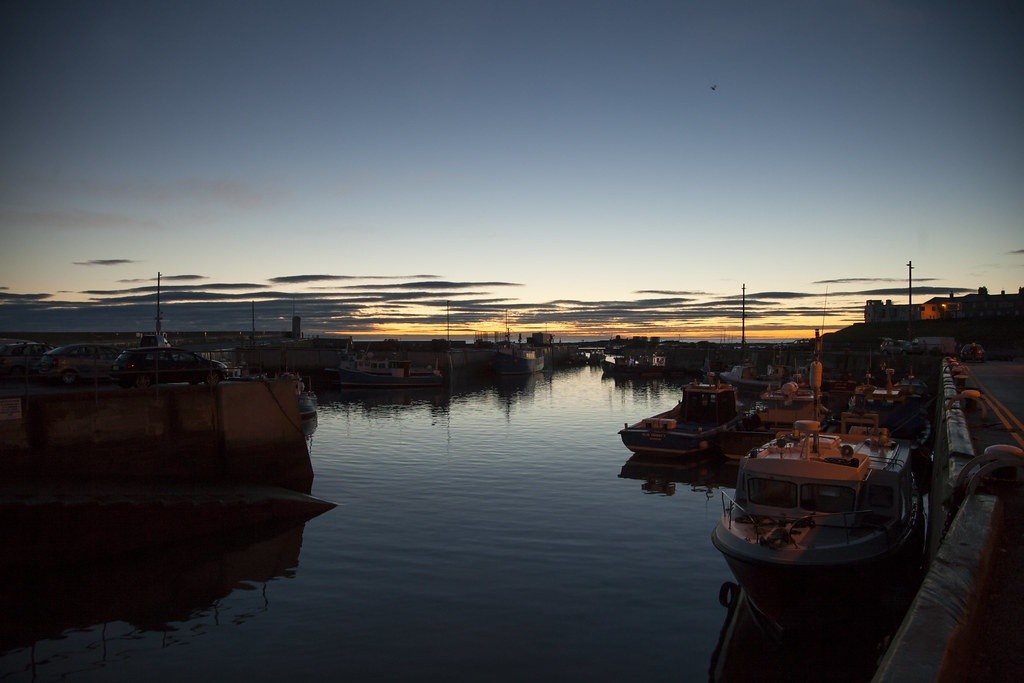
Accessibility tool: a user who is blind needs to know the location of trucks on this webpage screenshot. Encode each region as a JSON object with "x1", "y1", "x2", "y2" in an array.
[{"x1": 912, "y1": 335, "x2": 956, "y2": 355}]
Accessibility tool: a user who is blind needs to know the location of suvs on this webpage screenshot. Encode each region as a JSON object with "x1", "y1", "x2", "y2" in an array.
[{"x1": 880, "y1": 337, "x2": 920, "y2": 356}]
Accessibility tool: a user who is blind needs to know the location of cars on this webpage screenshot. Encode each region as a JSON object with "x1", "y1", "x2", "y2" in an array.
[
  {"x1": 0, "y1": 340, "x2": 58, "y2": 383},
  {"x1": 107, "y1": 346, "x2": 229, "y2": 390},
  {"x1": 959, "y1": 344, "x2": 986, "y2": 362},
  {"x1": 37, "y1": 340, "x2": 125, "y2": 386}
]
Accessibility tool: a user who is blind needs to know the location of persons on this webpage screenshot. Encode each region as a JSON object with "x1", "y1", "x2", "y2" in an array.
[{"x1": 970, "y1": 342, "x2": 977, "y2": 366}]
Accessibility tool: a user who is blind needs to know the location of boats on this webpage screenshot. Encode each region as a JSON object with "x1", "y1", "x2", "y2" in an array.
[
  {"x1": 707, "y1": 579, "x2": 921, "y2": 683},
  {"x1": 322, "y1": 339, "x2": 448, "y2": 391},
  {"x1": 491, "y1": 330, "x2": 544, "y2": 376},
  {"x1": 617, "y1": 329, "x2": 933, "y2": 637},
  {"x1": 139, "y1": 271, "x2": 317, "y2": 416}
]
